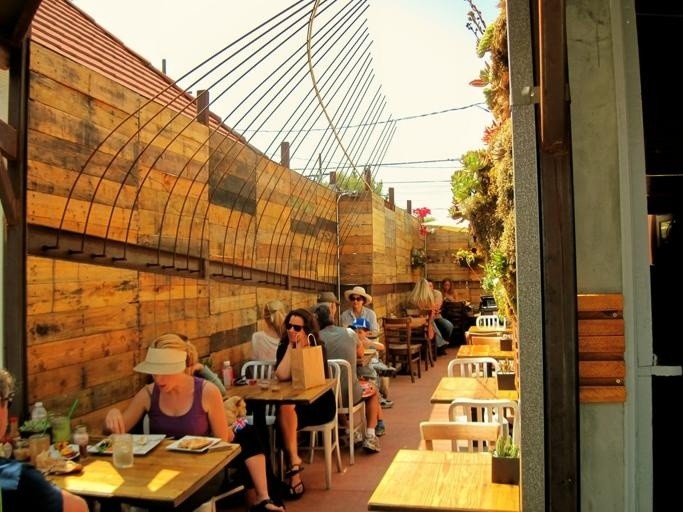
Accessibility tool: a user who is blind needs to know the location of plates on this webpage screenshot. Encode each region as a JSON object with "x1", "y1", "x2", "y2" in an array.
[
  {"x1": 47, "y1": 441, "x2": 91, "y2": 460},
  {"x1": 165, "y1": 433, "x2": 228, "y2": 453},
  {"x1": 87, "y1": 433, "x2": 167, "y2": 455}
]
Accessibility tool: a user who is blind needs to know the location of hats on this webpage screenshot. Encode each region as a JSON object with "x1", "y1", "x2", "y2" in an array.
[
  {"x1": 344, "y1": 286, "x2": 372, "y2": 304},
  {"x1": 347, "y1": 318, "x2": 370, "y2": 330},
  {"x1": 317, "y1": 291, "x2": 342, "y2": 306},
  {"x1": 131, "y1": 346, "x2": 188, "y2": 377}
]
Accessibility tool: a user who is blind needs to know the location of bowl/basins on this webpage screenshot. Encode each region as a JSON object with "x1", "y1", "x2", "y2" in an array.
[{"x1": 247, "y1": 376, "x2": 281, "y2": 394}]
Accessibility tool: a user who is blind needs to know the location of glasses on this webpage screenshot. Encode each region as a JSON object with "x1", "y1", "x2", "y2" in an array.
[
  {"x1": 350, "y1": 295, "x2": 363, "y2": 302},
  {"x1": 285, "y1": 323, "x2": 305, "y2": 332},
  {"x1": 0, "y1": 390, "x2": 16, "y2": 408}
]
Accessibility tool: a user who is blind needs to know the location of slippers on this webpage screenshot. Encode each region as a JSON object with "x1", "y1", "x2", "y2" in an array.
[
  {"x1": 248, "y1": 499, "x2": 286, "y2": 511},
  {"x1": 285, "y1": 463, "x2": 305, "y2": 479},
  {"x1": 287, "y1": 480, "x2": 305, "y2": 499}
]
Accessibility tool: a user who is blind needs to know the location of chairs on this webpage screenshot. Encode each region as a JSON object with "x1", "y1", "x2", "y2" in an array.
[
  {"x1": 130, "y1": 293, "x2": 520, "y2": 510},
  {"x1": 210, "y1": 358, "x2": 372, "y2": 492}
]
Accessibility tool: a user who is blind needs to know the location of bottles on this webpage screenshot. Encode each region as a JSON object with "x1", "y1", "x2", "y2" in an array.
[
  {"x1": 0, "y1": 415, "x2": 27, "y2": 460},
  {"x1": 73, "y1": 424, "x2": 88, "y2": 460},
  {"x1": 221, "y1": 360, "x2": 233, "y2": 389},
  {"x1": 31, "y1": 401, "x2": 47, "y2": 425}
]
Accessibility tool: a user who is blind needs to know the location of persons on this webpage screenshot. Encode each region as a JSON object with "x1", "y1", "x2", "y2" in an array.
[
  {"x1": 171, "y1": 333, "x2": 286, "y2": 512},
  {"x1": 273, "y1": 307, "x2": 335, "y2": 499},
  {"x1": 249, "y1": 300, "x2": 287, "y2": 363},
  {"x1": 408, "y1": 278, "x2": 460, "y2": 353},
  {"x1": 0, "y1": 367, "x2": 90, "y2": 512},
  {"x1": 312, "y1": 286, "x2": 397, "y2": 453},
  {"x1": 104, "y1": 334, "x2": 229, "y2": 512}
]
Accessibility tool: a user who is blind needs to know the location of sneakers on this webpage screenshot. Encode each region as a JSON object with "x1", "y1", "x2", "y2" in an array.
[
  {"x1": 362, "y1": 435, "x2": 383, "y2": 453},
  {"x1": 378, "y1": 397, "x2": 394, "y2": 409},
  {"x1": 376, "y1": 424, "x2": 385, "y2": 436},
  {"x1": 344, "y1": 430, "x2": 362, "y2": 448}
]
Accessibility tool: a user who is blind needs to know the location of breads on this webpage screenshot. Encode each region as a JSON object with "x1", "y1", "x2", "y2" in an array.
[
  {"x1": 61, "y1": 448, "x2": 75, "y2": 458},
  {"x1": 178, "y1": 439, "x2": 211, "y2": 450}
]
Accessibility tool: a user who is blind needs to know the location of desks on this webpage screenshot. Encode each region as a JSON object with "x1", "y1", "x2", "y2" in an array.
[{"x1": 365, "y1": 293, "x2": 520, "y2": 510}]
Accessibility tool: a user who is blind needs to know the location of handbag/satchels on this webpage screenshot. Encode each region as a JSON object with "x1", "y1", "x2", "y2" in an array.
[{"x1": 290, "y1": 334, "x2": 327, "y2": 388}]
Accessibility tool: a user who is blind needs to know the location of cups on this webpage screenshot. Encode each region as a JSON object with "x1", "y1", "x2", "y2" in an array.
[
  {"x1": 27, "y1": 434, "x2": 49, "y2": 463},
  {"x1": 50, "y1": 415, "x2": 70, "y2": 442},
  {"x1": 110, "y1": 434, "x2": 133, "y2": 466}
]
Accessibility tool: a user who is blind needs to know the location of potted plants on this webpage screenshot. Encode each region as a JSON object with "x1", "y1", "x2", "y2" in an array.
[{"x1": 18, "y1": 420, "x2": 52, "y2": 444}]
